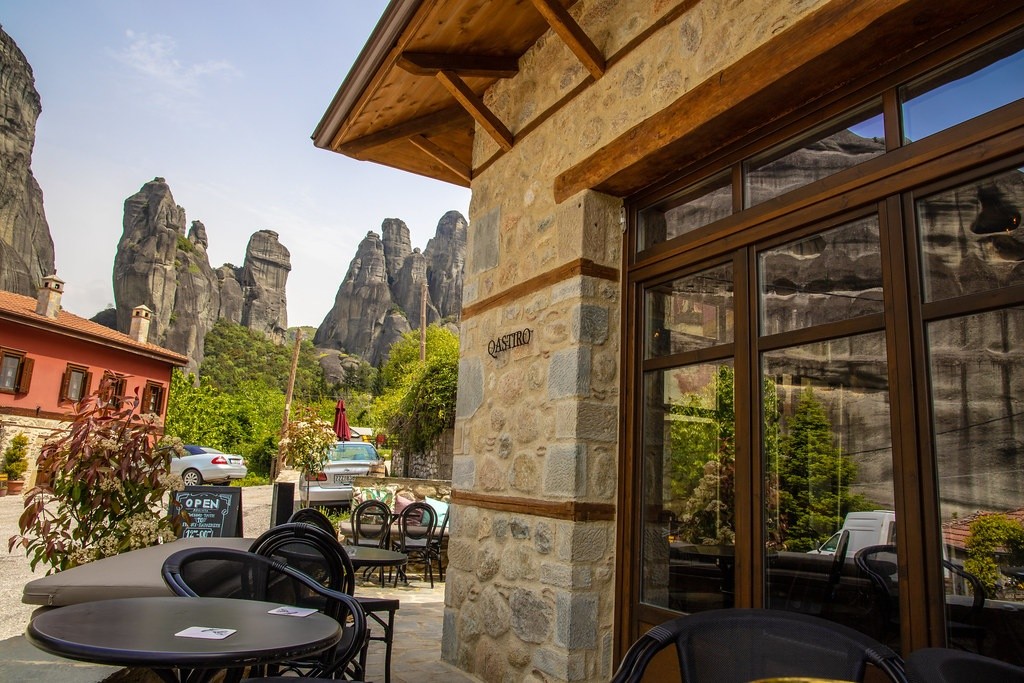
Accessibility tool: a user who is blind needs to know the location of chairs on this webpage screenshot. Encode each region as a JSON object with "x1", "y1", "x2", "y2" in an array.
[
  {"x1": 905, "y1": 648, "x2": 1024, "y2": 683},
  {"x1": 610, "y1": 609, "x2": 924, "y2": 683},
  {"x1": 353, "y1": 453, "x2": 366, "y2": 460},
  {"x1": 159, "y1": 500, "x2": 449, "y2": 683},
  {"x1": 669, "y1": 529, "x2": 986, "y2": 651}
]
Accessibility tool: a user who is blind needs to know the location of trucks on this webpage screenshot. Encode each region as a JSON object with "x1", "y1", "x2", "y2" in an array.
[{"x1": 805, "y1": 508, "x2": 950, "y2": 581}]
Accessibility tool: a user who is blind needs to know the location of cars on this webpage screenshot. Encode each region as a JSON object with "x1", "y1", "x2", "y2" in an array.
[
  {"x1": 298, "y1": 439, "x2": 389, "y2": 516},
  {"x1": 144, "y1": 445, "x2": 247, "y2": 485}
]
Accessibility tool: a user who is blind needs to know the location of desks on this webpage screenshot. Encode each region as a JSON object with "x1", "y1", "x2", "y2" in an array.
[
  {"x1": 274, "y1": 542, "x2": 407, "y2": 683},
  {"x1": 25, "y1": 597, "x2": 343, "y2": 683},
  {"x1": 341, "y1": 523, "x2": 449, "y2": 581}
]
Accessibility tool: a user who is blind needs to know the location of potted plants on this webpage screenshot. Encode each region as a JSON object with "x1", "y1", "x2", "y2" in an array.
[{"x1": 3, "y1": 432, "x2": 30, "y2": 495}]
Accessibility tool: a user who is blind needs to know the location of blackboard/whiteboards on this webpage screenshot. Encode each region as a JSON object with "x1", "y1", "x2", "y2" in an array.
[{"x1": 167, "y1": 487, "x2": 241, "y2": 537}]
